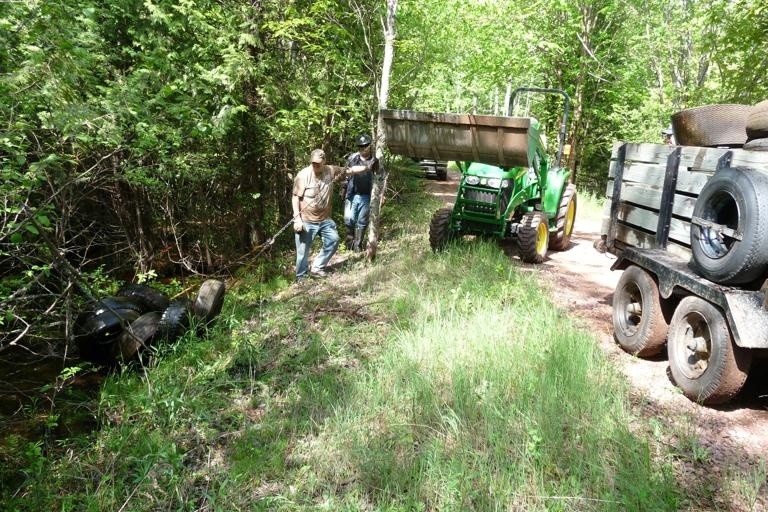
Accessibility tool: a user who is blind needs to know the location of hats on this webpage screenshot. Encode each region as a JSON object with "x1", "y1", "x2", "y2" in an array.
[
  {"x1": 311, "y1": 148, "x2": 326, "y2": 164},
  {"x1": 358, "y1": 134, "x2": 371, "y2": 147}
]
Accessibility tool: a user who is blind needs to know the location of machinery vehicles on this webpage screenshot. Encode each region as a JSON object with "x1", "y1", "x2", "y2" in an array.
[{"x1": 377, "y1": 84, "x2": 582, "y2": 265}]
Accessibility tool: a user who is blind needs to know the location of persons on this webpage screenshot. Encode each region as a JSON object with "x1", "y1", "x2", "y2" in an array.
[
  {"x1": 292, "y1": 149, "x2": 375, "y2": 284},
  {"x1": 344, "y1": 135, "x2": 374, "y2": 254}
]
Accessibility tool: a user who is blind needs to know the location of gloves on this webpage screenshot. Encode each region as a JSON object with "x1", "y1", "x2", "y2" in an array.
[
  {"x1": 365, "y1": 157, "x2": 378, "y2": 172},
  {"x1": 293, "y1": 218, "x2": 306, "y2": 234}
]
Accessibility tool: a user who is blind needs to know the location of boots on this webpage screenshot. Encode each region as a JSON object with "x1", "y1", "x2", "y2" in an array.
[
  {"x1": 353, "y1": 227, "x2": 364, "y2": 254},
  {"x1": 345, "y1": 224, "x2": 353, "y2": 251}
]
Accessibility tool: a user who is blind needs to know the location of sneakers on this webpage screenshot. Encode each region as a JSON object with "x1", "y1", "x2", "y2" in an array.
[{"x1": 311, "y1": 268, "x2": 327, "y2": 277}]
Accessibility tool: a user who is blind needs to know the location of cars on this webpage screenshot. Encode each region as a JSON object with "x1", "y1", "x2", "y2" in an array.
[{"x1": 399, "y1": 150, "x2": 448, "y2": 183}]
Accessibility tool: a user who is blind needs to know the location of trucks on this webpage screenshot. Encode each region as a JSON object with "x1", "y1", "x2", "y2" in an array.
[{"x1": 591, "y1": 99, "x2": 768, "y2": 408}]
